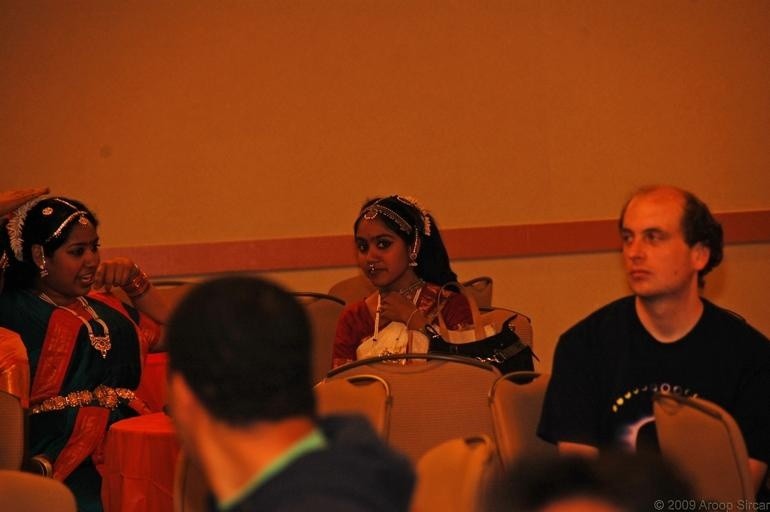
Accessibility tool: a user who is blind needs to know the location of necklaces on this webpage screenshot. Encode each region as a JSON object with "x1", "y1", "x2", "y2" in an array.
[{"x1": 34, "y1": 290, "x2": 112, "y2": 359}]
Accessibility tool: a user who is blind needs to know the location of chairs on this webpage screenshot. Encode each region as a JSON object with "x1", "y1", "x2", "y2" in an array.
[{"x1": 123, "y1": 273, "x2": 764, "y2": 512}]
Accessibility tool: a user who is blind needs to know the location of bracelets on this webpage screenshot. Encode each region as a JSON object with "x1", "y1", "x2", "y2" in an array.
[{"x1": 123, "y1": 260, "x2": 152, "y2": 300}]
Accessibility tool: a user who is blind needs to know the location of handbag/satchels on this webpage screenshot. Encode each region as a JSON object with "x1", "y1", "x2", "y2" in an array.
[{"x1": 425, "y1": 281, "x2": 535, "y2": 384}]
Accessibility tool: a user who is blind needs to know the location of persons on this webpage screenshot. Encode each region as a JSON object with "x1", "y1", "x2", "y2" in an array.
[
  {"x1": 333, "y1": 195, "x2": 475, "y2": 370},
  {"x1": 538, "y1": 184, "x2": 770, "y2": 512},
  {"x1": 0, "y1": 195, "x2": 172, "y2": 512},
  {"x1": 162, "y1": 274, "x2": 412, "y2": 512}
]
